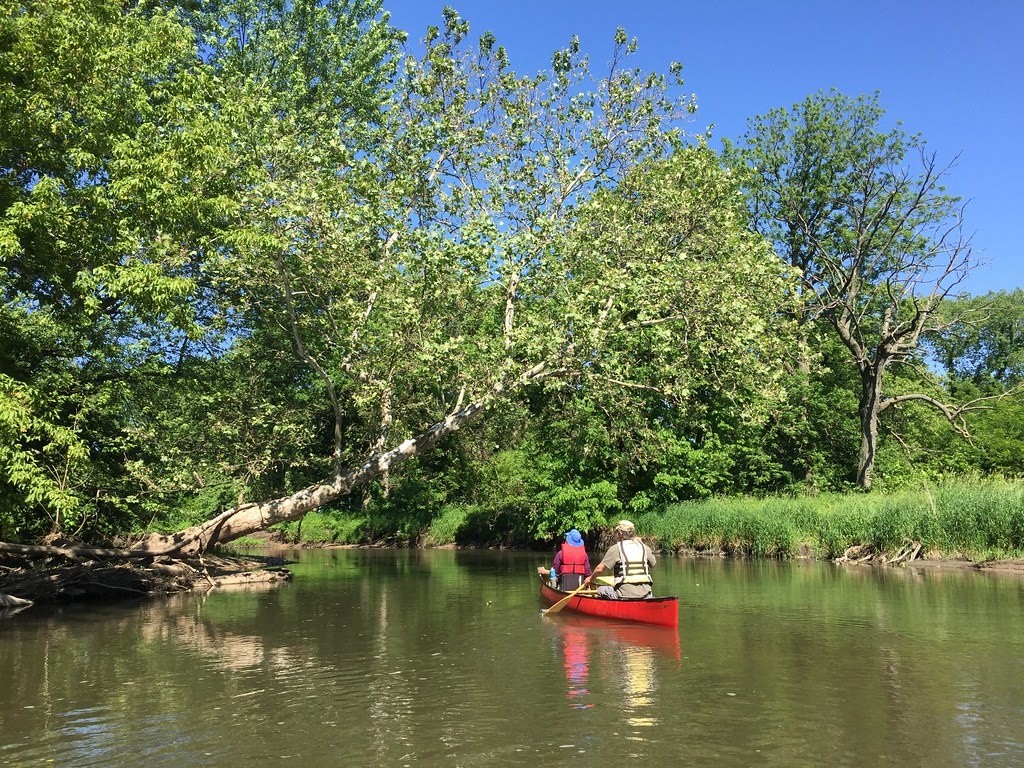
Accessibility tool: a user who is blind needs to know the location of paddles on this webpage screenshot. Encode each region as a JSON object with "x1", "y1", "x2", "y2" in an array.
[{"x1": 543, "y1": 583, "x2": 587, "y2": 614}]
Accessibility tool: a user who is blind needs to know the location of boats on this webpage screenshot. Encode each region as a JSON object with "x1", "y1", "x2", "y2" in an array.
[
  {"x1": 550, "y1": 608, "x2": 682, "y2": 668},
  {"x1": 538, "y1": 567, "x2": 678, "y2": 629}
]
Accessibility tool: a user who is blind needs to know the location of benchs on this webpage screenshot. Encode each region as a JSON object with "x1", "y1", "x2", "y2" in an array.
[{"x1": 564, "y1": 590, "x2": 598, "y2": 598}]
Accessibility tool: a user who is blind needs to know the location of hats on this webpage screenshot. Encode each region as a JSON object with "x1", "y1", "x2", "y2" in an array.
[
  {"x1": 616, "y1": 520, "x2": 634, "y2": 531},
  {"x1": 565, "y1": 529, "x2": 583, "y2": 546}
]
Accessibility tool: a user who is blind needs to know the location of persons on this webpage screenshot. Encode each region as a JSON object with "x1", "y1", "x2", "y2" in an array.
[
  {"x1": 584, "y1": 520, "x2": 657, "y2": 600},
  {"x1": 537, "y1": 529, "x2": 591, "y2": 591}
]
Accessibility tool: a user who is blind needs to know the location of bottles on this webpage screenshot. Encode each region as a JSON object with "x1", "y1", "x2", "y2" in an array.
[{"x1": 549, "y1": 567, "x2": 555, "y2": 580}]
